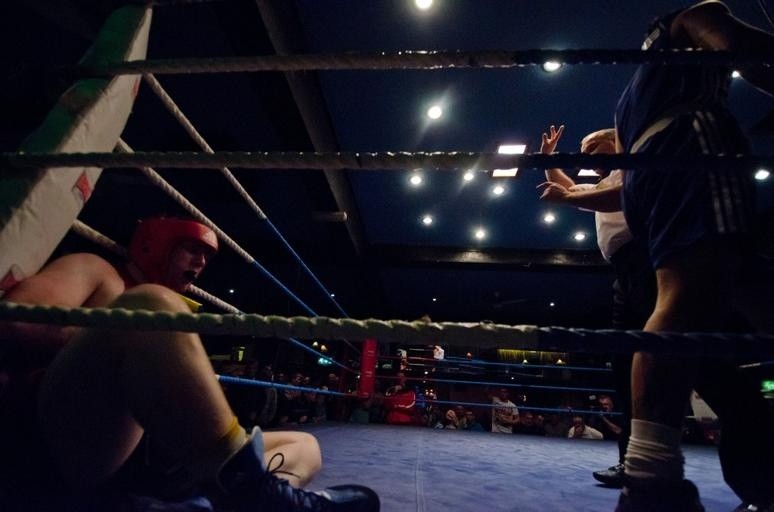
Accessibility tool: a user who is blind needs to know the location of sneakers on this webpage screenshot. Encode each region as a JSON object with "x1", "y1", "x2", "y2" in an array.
[
  {"x1": 615, "y1": 471, "x2": 705, "y2": 512},
  {"x1": 208, "y1": 428, "x2": 380, "y2": 511}
]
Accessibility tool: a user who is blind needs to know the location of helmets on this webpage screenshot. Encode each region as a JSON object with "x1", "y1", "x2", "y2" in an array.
[{"x1": 126, "y1": 216, "x2": 219, "y2": 292}]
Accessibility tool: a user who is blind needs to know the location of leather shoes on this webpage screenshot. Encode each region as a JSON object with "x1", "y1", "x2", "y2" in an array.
[{"x1": 593, "y1": 462, "x2": 626, "y2": 483}]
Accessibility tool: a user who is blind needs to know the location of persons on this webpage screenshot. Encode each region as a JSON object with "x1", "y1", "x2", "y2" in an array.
[
  {"x1": 534, "y1": 121, "x2": 696, "y2": 488},
  {"x1": 0, "y1": 212, "x2": 382, "y2": 512},
  {"x1": 1, "y1": 358, "x2": 622, "y2": 511},
  {"x1": 612, "y1": 0, "x2": 774, "y2": 512}
]
